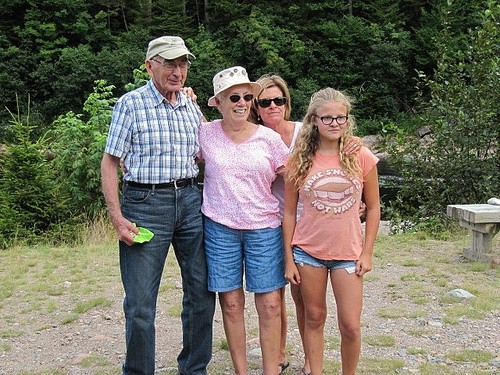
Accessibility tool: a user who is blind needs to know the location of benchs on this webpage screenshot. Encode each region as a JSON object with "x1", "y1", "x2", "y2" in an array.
[{"x1": 447, "y1": 203, "x2": 500, "y2": 264}]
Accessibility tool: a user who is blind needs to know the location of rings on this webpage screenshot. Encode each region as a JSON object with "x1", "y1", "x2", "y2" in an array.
[{"x1": 353, "y1": 143, "x2": 357, "y2": 147}]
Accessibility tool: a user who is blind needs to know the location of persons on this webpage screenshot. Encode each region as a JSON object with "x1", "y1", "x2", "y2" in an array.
[
  {"x1": 277, "y1": 87, "x2": 380, "y2": 375},
  {"x1": 101, "y1": 36, "x2": 216, "y2": 375},
  {"x1": 254, "y1": 74, "x2": 362, "y2": 375},
  {"x1": 180, "y1": 65, "x2": 366, "y2": 375}
]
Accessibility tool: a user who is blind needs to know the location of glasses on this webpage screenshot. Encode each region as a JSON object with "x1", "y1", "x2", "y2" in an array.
[
  {"x1": 314, "y1": 113, "x2": 349, "y2": 126},
  {"x1": 223, "y1": 94, "x2": 254, "y2": 103},
  {"x1": 257, "y1": 97, "x2": 287, "y2": 108},
  {"x1": 151, "y1": 58, "x2": 192, "y2": 70}
]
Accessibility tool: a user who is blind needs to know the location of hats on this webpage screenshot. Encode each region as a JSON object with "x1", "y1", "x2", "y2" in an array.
[
  {"x1": 207, "y1": 66, "x2": 261, "y2": 106},
  {"x1": 146, "y1": 36, "x2": 196, "y2": 61}
]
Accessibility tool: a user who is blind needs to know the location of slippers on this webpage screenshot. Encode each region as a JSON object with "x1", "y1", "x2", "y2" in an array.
[{"x1": 278, "y1": 361, "x2": 289, "y2": 374}]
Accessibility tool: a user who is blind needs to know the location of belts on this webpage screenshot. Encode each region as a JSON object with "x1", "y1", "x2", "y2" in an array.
[{"x1": 128, "y1": 177, "x2": 198, "y2": 188}]
